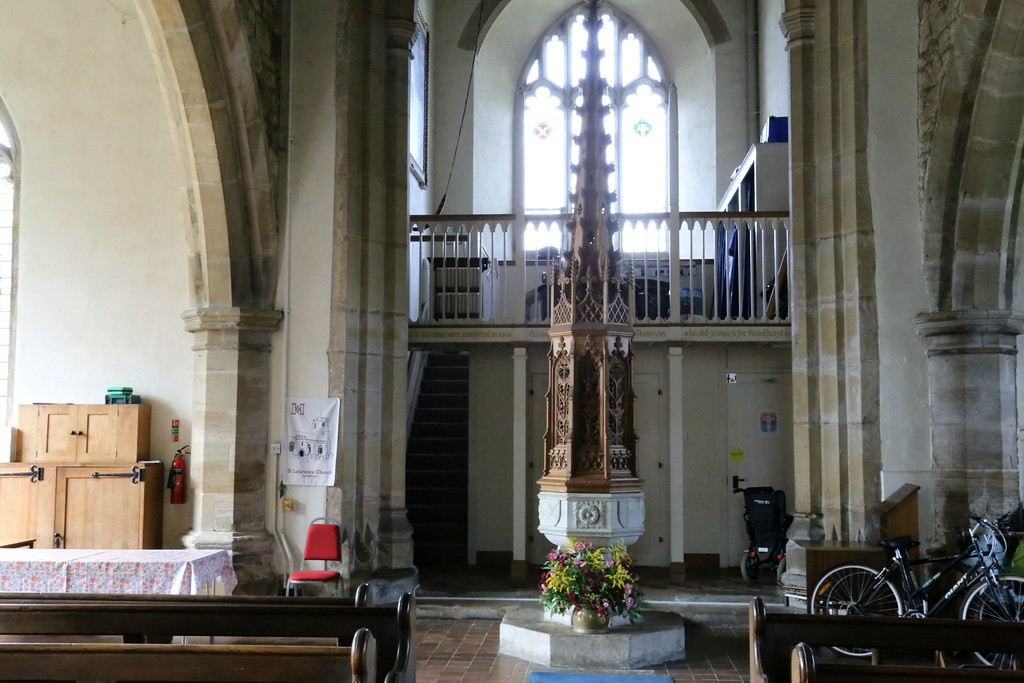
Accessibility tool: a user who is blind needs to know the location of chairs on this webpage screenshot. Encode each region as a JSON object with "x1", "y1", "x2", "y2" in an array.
[{"x1": 285, "y1": 518, "x2": 344, "y2": 598}]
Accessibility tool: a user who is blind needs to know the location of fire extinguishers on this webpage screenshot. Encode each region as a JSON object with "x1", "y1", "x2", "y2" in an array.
[{"x1": 167, "y1": 445, "x2": 191, "y2": 504}]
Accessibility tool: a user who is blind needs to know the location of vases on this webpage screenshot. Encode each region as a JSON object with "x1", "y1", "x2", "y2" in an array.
[{"x1": 572, "y1": 603, "x2": 609, "y2": 635}]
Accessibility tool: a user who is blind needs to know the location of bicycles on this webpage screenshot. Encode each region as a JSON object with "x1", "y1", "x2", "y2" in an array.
[{"x1": 806, "y1": 510, "x2": 1024, "y2": 669}]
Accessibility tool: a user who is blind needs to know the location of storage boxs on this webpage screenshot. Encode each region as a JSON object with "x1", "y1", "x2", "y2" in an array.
[{"x1": 760, "y1": 116, "x2": 788, "y2": 142}]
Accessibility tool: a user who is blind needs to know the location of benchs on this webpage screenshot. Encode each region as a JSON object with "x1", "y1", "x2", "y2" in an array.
[
  {"x1": 747, "y1": 596, "x2": 1024, "y2": 683},
  {"x1": 0, "y1": 584, "x2": 418, "y2": 683}
]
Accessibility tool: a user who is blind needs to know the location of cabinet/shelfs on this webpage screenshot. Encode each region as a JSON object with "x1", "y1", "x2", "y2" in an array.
[
  {"x1": 426, "y1": 254, "x2": 489, "y2": 320},
  {"x1": 15, "y1": 404, "x2": 152, "y2": 464},
  {"x1": 0, "y1": 463, "x2": 165, "y2": 551}
]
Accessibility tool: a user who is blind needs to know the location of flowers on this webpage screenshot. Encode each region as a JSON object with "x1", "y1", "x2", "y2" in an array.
[{"x1": 540, "y1": 539, "x2": 647, "y2": 624}]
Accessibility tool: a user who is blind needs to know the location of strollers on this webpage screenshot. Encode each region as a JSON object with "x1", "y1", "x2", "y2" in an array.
[{"x1": 737, "y1": 486, "x2": 794, "y2": 587}]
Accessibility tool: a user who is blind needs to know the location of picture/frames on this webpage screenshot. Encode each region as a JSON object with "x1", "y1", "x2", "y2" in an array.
[{"x1": 409, "y1": 5, "x2": 433, "y2": 190}]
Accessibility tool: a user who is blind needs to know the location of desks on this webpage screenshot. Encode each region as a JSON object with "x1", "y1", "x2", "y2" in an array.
[{"x1": 0, "y1": 548, "x2": 237, "y2": 645}]
